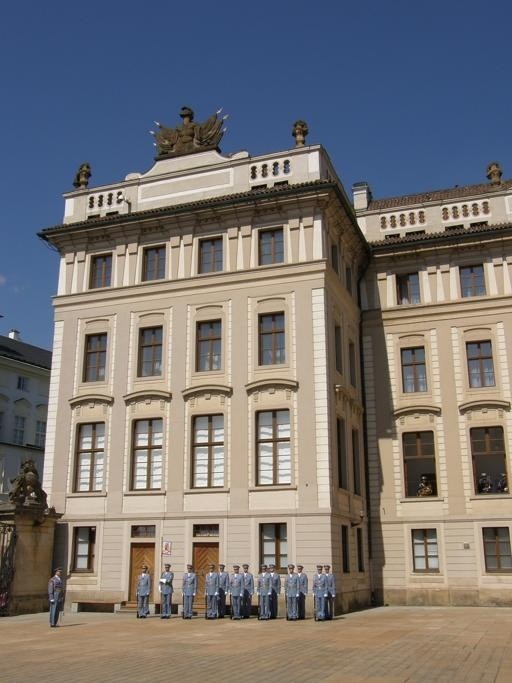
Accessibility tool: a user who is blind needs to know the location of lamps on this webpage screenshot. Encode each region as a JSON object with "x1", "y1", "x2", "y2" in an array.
[
  {"x1": 33, "y1": 508, "x2": 57, "y2": 525},
  {"x1": 352, "y1": 511, "x2": 365, "y2": 527}
]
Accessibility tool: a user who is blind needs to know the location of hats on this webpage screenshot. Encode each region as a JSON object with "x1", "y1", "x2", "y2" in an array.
[
  {"x1": 186, "y1": 563, "x2": 193, "y2": 570},
  {"x1": 317, "y1": 564, "x2": 331, "y2": 571},
  {"x1": 140, "y1": 564, "x2": 148, "y2": 569},
  {"x1": 232, "y1": 564, "x2": 250, "y2": 569},
  {"x1": 208, "y1": 564, "x2": 225, "y2": 569},
  {"x1": 259, "y1": 563, "x2": 276, "y2": 570},
  {"x1": 163, "y1": 563, "x2": 171, "y2": 567},
  {"x1": 288, "y1": 563, "x2": 304, "y2": 570},
  {"x1": 54, "y1": 565, "x2": 64, "y2": 573}
]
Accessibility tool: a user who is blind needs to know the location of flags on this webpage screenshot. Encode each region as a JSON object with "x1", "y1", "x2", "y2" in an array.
[{"x1": 162, "y1": 540, "x2": 172, "y2": 556}]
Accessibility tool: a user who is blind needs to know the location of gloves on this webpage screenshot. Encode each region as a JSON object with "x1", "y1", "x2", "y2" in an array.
[
  {"x1": 323, "y1": 593, "x2": 336, "y2": 598},
  {"x1": 239, "y1": 594, "x2": 244, "y2": 598},
  {"x1": 182, "y1": 592, "x2": 196, "y2": 597},
  {"x1": 214, "y1": 590, "x2": 229, "y2": 597},
  {"x1": 296, "y1": 593, "x2": 300, "y2": 598},
  {"x1": 256, "y1": 592, "x2": 272, "y2": 597},
  {"x1": 158, "y1": 577, "x2": 166, "y2": 583},
  {"x1": 50, "y1": 599, "x2": 55, "y2": 603},
  {"x1": 159, "y1": 587, "x2": 162, "y2": 593}
]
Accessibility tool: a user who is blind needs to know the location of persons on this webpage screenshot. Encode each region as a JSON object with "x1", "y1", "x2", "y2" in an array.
[
  {"x1": 136, "y1": 565, "x2": 151, "y2": 618},
  {"x1": 417, "y1": 476, "x2": 433, "y2": 496},
  {"x1": 496, "y1": 473, "x2": 508, "y2": 494},
  {"x1": 47, "y1": 567, "x2": 65, "y2": 627},
  {"x1": 204, "y1": 563, "x2": 336, "y2": 620},
  {"x1": 158, "y1": 564, "x2": 174, "y2": 619},
  {"x1": 476, "y1": 472, "x2": 493, "y2": 494},
  {"x1": 181, "y1": 564, "x2": 197, "y2": 619}
]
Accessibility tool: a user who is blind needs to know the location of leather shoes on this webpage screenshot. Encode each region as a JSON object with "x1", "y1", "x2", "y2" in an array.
[{"x1": 138, "y1": 615, "x2": 324, "y2": 622}]
